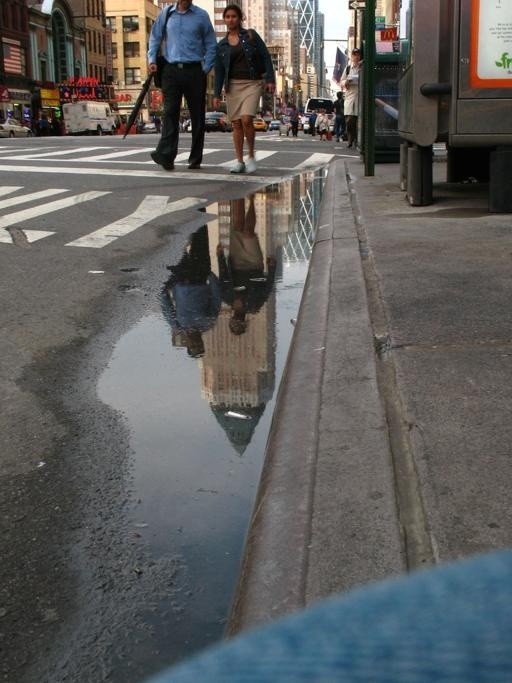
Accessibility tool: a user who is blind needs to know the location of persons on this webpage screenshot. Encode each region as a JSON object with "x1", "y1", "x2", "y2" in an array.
[
  {"x1": 211, "y1": 192, "x2": 277, "y2": 336},
  {"x1": 211, "y1": 4, "x2": 276, "y2": 175},
  {"x1": 153, "y1": 205, "x2": 221, "y2": 359},
  {"x1": 297, "y1": 110, "x2": 306, "y2": 131},
  {"x1": 340, "y1": 47, "x2": 364, "y2": 147},
  {"x1": 288, "y1": 104, "x2": 298, "y2": 136},
  {"x1": 146, "y1": 0, "x2": 218, "y2": 171},
  {"x1": 330, "y1": 90, "x2": 348, "y2": 142},
  {"x1": 314, "y1": 107, "x2": 329, "y2": 141},
  {"x1": 308, "y1": 109, "x2": 318, "y2": 136}
]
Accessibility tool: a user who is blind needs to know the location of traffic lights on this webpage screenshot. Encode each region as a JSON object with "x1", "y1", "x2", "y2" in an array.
[
  {"x1": 294, "y1": 84, "x2": 298, "y2": 91},
  {"x1": 297, "y1": 84, "x2": 301, "y2": 92}
]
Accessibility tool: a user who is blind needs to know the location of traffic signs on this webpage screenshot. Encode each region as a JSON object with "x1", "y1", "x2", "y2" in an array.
[{"x1": 348, "y1": 0, "x2": 377, "y2": 10}]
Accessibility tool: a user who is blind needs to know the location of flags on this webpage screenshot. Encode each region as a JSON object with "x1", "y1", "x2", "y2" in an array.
[{"x1": 332, "y1": 45, "x2": 348, "y2": 85}]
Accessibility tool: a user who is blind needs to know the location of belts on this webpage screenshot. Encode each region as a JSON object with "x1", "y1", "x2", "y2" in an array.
[{"x1": 169, "y1": 62, "x2": 201, "y2": 69}]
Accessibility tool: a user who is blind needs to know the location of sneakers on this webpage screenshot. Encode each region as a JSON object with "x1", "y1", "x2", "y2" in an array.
[
  {"x1": 244, "y1": 157, "x2": 257, "y2": 174},
  {"x1": 230, "y1": 162, "x2": 245, "y2": 173}
]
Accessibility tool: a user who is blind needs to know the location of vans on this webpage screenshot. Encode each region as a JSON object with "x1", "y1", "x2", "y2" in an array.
[{"x1": 303, "y1": 96, "x2": 336, "y2": 135}]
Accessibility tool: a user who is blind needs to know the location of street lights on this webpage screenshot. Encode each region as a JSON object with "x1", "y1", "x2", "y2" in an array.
[{"x1": 320, "y1": 38, "x2": 348, "y2": 48}]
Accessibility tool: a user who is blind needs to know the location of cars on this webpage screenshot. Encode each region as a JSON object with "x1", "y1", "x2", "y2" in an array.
[
  {"x1": 112, "y1": 110, "x2": 303, "y2": 134},
  {"x1": 1, "y1": 118, "x2": 33, "y2": 138}
]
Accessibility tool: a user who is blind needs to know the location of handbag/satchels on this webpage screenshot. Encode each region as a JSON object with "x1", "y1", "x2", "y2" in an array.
[{"x1": 153, "y1": 56, "x2": 166, "y2": 88}]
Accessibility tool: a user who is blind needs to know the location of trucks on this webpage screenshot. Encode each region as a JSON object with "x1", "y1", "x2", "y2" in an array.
[{"x1": 62, "y1": 100, "x2": 116, "y2": 135}]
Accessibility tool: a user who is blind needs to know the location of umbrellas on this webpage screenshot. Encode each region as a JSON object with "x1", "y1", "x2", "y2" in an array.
[{"x1": 122, "y1": 64, "x2": 156, "y2": 138}]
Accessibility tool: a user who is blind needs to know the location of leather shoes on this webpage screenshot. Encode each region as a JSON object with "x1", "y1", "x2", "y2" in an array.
[
  {"x1": 188, "y1": 163, "x2": 200, "y2": 169},
  {"x1": 150, "y1": 151, "x2": 174, "y2": 170}
]
